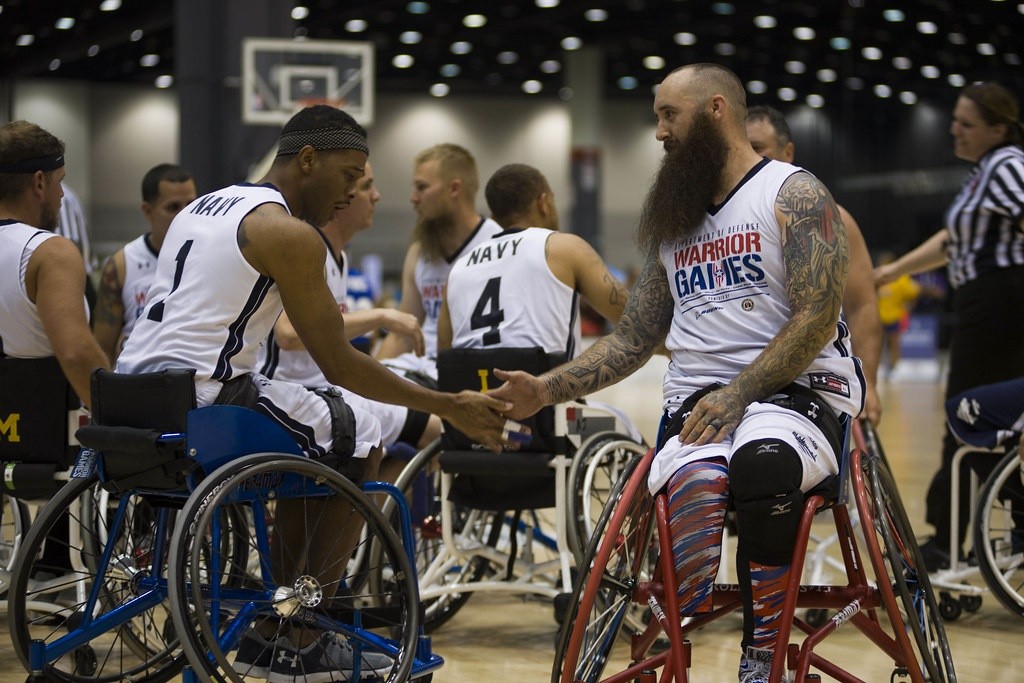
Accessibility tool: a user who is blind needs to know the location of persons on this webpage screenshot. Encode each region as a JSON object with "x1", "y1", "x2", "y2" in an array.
[
  {"x1": 870, "y1": 81, "x2": 1024, "y2": 577},
  {"x1": 869, "y1": 243, "x2": 953, "y2": 394},
  {"x1": 481, "y1": 59, "x2": 868, "y2": 682},
  {"x1": 48, "y1": 170, "x2": 100, "y2": 337},
  {"x1": 93, "y1": 162, "x2": 196, "y2": 374},
  {"x1": 105, "y1": 100, "x2": 534, "y2": 681},
  {"x1": 0, "y1": 118, "x2": 151, "y2": 578},
  {"x1": 272, "y1": 147, "x2": 654, "y2": 518},
  {"x1": 741, "y1": 101, "x2": 886, "y2": 426}
]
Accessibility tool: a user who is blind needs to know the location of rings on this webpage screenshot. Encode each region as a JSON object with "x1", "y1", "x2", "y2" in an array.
[{"x1": 710, "y1": 420, "x2": 721, "y2": 431}]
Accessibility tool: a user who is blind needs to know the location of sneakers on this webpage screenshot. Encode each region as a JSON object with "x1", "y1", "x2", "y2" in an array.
[
  {"x1": 267, "y1": 628, "x2": 393, "y2": 683},
  {"x1": 230, "y1": 625, "x2": 272, "y2": 680}
]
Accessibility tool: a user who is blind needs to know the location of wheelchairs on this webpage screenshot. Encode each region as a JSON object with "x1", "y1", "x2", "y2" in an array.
[{"x1": 0, "y1": 348, "x2": 1024, "y2": 683}]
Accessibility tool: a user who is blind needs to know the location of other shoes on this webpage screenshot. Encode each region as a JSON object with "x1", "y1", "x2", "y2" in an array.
[
  {"x1": 738, "y1": 644, "x2": 789, "y2": 683},
  {"x1": 919, "y1": 531, "x2": 964, "y2": 572},
  {"x1": 965, "y1": 526, "x2": 1024, "y2": 567}
]
